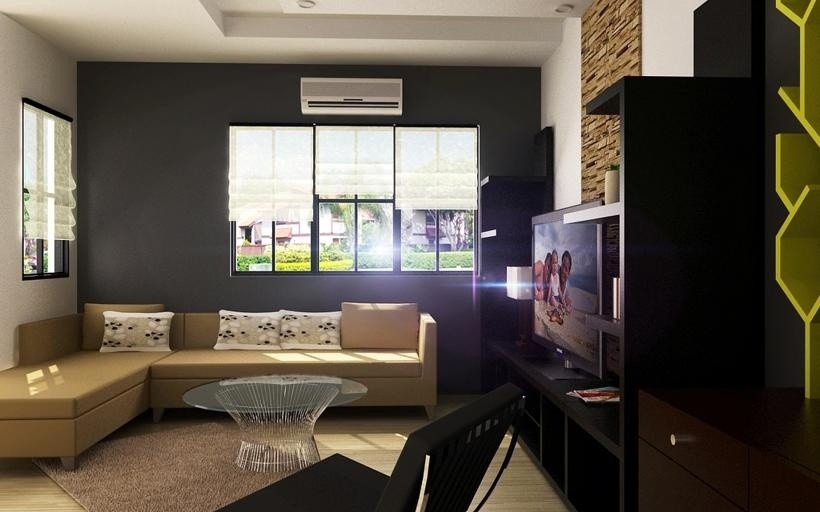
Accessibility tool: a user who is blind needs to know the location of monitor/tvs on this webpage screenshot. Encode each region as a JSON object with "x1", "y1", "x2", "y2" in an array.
[{"x1": 530, "y1": 200, "x2": 608, "y2": 383}]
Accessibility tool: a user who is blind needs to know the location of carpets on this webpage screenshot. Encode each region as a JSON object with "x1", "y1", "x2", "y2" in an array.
[{"x1": 31, "y1": 421, "x2": 313, "y2": 512}]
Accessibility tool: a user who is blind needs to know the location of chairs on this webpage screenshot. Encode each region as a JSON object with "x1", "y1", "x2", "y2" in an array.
[{"x1": 212, "y1": 383, "x2": 526, "y2": 512}]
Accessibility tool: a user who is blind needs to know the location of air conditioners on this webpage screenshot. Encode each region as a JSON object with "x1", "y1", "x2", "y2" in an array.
[{"x1": 300, "y1": 77, "x2": 404, "y2": 117}]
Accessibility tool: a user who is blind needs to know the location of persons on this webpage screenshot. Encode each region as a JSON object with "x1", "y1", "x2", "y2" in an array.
[
  {"x1": 559, "y1": 250, "x2": 573, "y2": 308},
  {"x1": 533, "y1": 251, "x2": 550, "y2": 302},
  {"x1": 544, "y1": 249, "x2": 574, "y2": 315}
]
